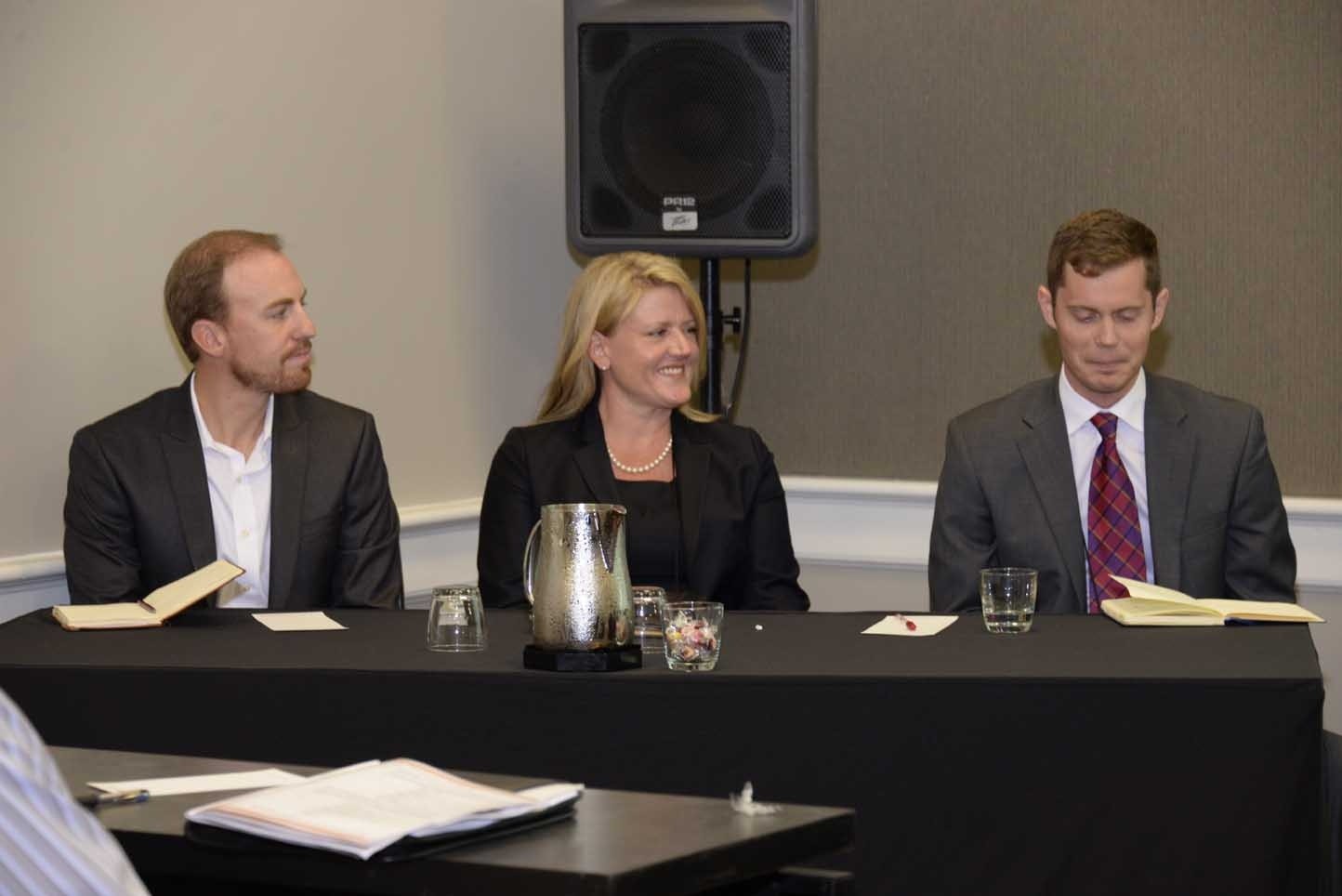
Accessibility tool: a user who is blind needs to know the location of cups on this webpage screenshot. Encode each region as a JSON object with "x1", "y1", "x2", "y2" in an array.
[
  {"x1": 425, "y1": 584, "x2": 486, "y2": 653},
  {"x1": 628, "y1": 584, "x2": 671, "y2": 656},
  {"x1": 979, "y1": 568, "x2": 1038, "y2": 634},
  {"x1": 662, "y1": 600, "x2": 724, "y2": 672}
]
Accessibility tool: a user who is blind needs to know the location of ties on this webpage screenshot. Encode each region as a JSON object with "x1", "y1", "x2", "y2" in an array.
[{"x1": 1082, "y1": 413, "x2": 1149, "y2": 615}]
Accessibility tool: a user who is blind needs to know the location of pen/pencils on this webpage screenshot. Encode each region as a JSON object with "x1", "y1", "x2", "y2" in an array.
[
  {"x1": 137, "y1": 598, "x2": 156, "y2": 615},
  {"x1": 74, "y1": 789, "x2": 151, "y2": 812},
  {"x1": 895, "y1": 613, "x2": 917, "y2": 631}
]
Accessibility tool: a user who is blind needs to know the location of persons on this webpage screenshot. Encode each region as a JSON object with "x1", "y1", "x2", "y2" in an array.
[
  {"x1": 927, "y1": 215, "x2": 1299, "y2": 616},
  {"x1": 476, "y1": 254, "x2": 810, "y2": 610},
  {"x1": 62, "y1": 230, "x2": 406, "y2": 615}
]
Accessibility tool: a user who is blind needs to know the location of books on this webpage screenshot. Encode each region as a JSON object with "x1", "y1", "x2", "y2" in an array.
[
  {"x1": 51, "y1": 558, "x2": 246, "y2": 631},
  {"x1": 1099, "y1": 573, "x2": 1326, "y2": 627},
  {"x1": 186, "y1": 756, "x2": 586, "y2": 863}
]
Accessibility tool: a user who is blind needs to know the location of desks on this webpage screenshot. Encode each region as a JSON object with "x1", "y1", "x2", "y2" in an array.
[
  {"x1": 43, "y1": 745, "x2": 857, "y2": 896},
  {"x1": 0, "y1": 602, "x2": 1324, "y2": 896}
]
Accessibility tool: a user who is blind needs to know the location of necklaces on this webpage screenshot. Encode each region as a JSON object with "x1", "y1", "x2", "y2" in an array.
[{"x1": 606, "y1": 438, "x2": 674, "y2": 473}]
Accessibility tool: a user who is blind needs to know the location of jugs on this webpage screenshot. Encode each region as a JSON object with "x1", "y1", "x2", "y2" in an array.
[{"x1": 522, "y1": 501, "x2": 645, "y2": 669}]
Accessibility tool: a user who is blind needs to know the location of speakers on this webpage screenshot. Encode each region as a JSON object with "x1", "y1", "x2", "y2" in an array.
[{"x1": 561, "y1": 0, "x2": 820, "y2": 261}]
